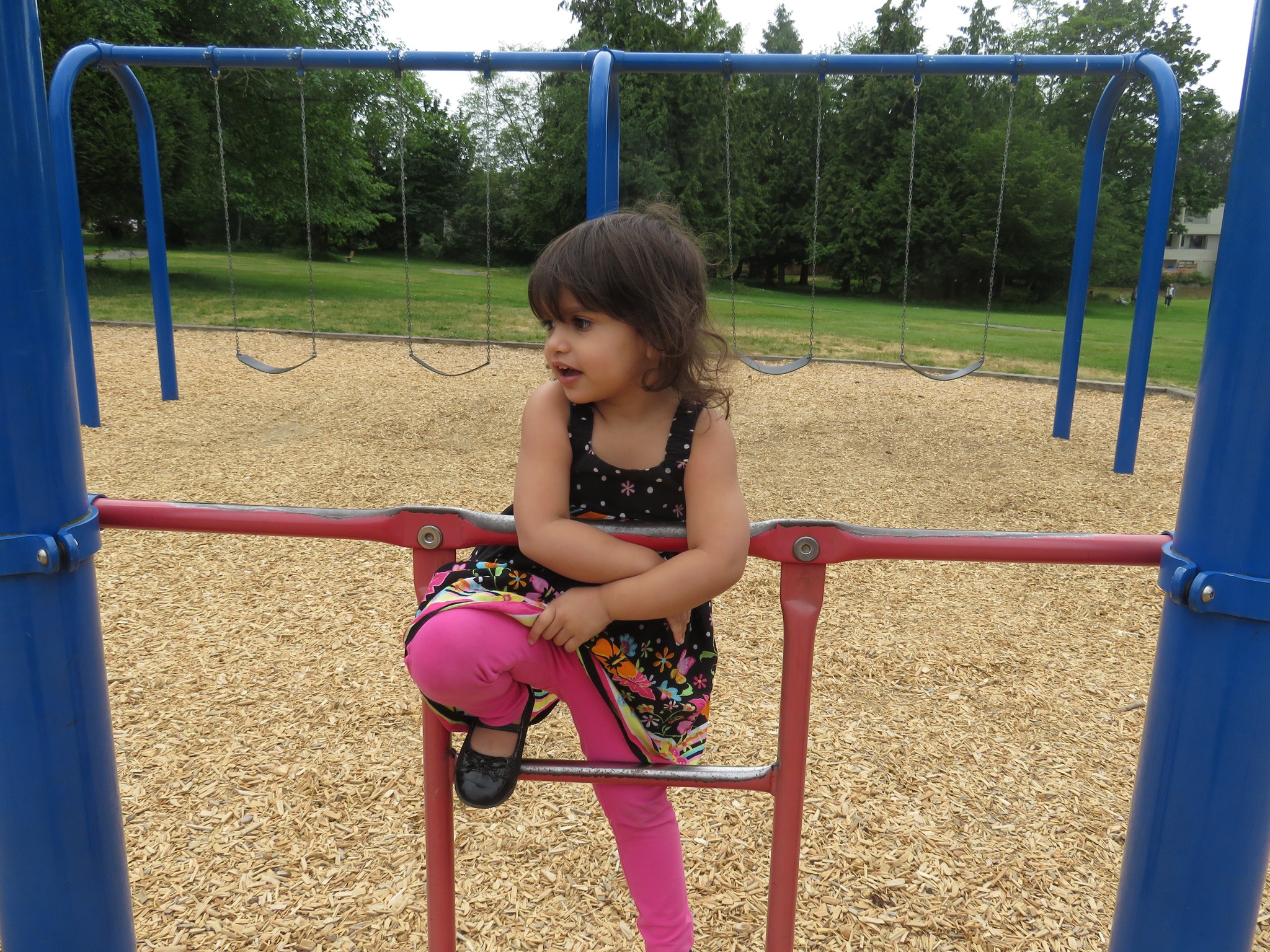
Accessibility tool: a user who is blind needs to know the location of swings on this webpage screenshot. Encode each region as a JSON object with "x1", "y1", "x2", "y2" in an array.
[
  {"x1": 209, "y1": 68, "x2": 319, "y2": 375},
  {"x1": 722, "y1": 77, "x2": 824, "y2": 376},
  {"x1": 898, "y1": 77, "x2": 1018, "y2": 382},
  {"x1": 393, "y1": 75, "x2": 492, "y2": 377}
]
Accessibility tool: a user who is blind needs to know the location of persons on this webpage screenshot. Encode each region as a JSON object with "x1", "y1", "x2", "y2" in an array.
[
  {"x1": 1163, "y1": 284, "x2": 1175, "y2": 307},
  {"x1": 403, "y1": 200, "x2": 749, "y2": 952},
  {"x1": 1131, "y1": 287, "x2": 1137, "y2": 304}
]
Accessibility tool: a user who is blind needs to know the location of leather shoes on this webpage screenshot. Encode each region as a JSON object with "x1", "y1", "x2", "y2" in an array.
[{"x1": 455, "y1": 683, "x2": 536, "y2": 808}]
